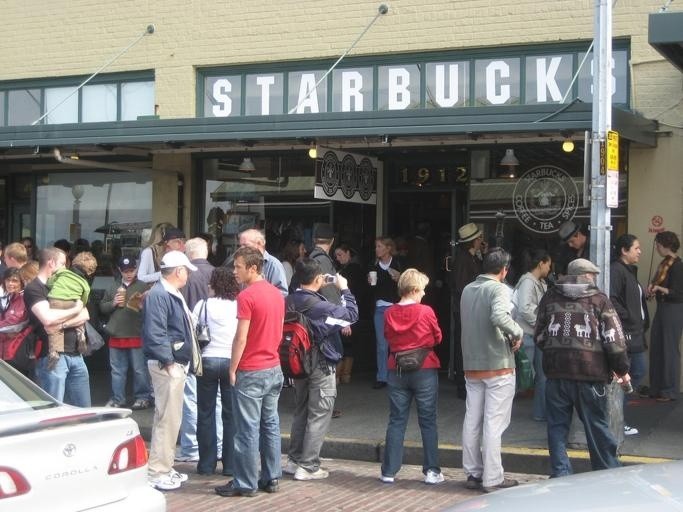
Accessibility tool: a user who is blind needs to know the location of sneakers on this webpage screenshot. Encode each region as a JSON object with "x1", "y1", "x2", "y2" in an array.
[
  {"x1": 373, "y1": 381, "x2": 386, "y2": 388},
  {"x1": 46, "y1": 352, "x2": 59, "y2": 371},
  {"x1": 104, "y1": 400, "x2": 121, "y2": 408},
  {"x1": 623, "y1": 425, "x2": 638, "y2": 436},
  {"x1": 76, "y1": 335, "x2": 88, "y2": 356},
  {"x1": 132, "y1": 398, "x2": 149, "y2": 409}
]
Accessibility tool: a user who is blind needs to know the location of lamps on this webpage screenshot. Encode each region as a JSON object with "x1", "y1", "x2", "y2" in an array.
[
  {"x1": 239, "y1": 157, "x2": 254, "y2": 171},
  {"x1": 498, "y1": 149, "x2": 519, "y2": 167}
]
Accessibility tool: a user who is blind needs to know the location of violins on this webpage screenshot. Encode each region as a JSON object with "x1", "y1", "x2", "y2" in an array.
[{"x1": 648, "y1": 256, "x2": 674, "y2": 301}]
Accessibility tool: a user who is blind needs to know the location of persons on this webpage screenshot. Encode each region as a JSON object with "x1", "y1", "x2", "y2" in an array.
[
  {"x1": 639, "y1": 231, "x2": 683, "y2": 402},
  {"x1": 609, "y1": 235, "x2": 651, "y2": 435},
  {"x1": 1, "y1": 222, "x2": 589, "y2": 496},
  {"x1": 533, "y1": 258, "x2": 634, "y2": 479}
]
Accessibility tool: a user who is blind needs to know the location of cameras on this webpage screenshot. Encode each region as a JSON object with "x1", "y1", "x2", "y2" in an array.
[{"x1": 326, "y1": 276, "x2": 336, "y2": 284}]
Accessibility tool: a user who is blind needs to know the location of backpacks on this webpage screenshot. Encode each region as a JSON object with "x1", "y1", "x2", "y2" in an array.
[{"x1": 277, "y1": 295, "x2": 327, "y2": 379}]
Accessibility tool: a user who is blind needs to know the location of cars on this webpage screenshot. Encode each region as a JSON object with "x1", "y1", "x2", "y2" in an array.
[
  {"x1": 0, "y1": 358, "x2": 166, "y2": 512},
  {"x1": 434, "y1": 458, "x2": 681, "y2": 510}
]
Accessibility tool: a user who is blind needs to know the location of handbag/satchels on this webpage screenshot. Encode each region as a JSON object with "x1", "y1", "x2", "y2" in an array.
[
  {"x1": 517, "y1": 345, "x2": 534, "y2": 391},
  {"x1": 196, "y1": 322, "x2": 211, "y2": 349},
  {"x1": 394, "y1": 346, "x2": 427, "y2": 372},
  {"x1": 6, "y1": 326, "x2": 37, "y2": 373}
]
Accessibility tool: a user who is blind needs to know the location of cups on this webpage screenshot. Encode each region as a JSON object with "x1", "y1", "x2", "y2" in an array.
[
  {"x1": 369, "y1": 271, "x2": 378, "y2": 287},
  {"x1": 117, "y1": 286, "x2": 126, "y2": 307}
]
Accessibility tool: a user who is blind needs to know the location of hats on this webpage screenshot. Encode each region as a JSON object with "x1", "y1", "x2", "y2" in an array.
[
  {"x1": 117, "y1": 255, "x2": 137, "y2": 272},
  {"x1": 160, "y1": 250, "x2": 198, "y2": 272},
  {"x1": 558, "y1": 221, "x2": 582, "y2": 246},
  {"x1": 458, "y1": 222, "x2": 483, "y2": 243},
  {"x1": 313, "y1": 223, "x2": 334, "y2": 239},
  {"x1": 163, "y1": 228, "x2": 186, "y2": 241},
  {"x1": 568, "y1": 257, "x2": 602, "y2": 275}
]
